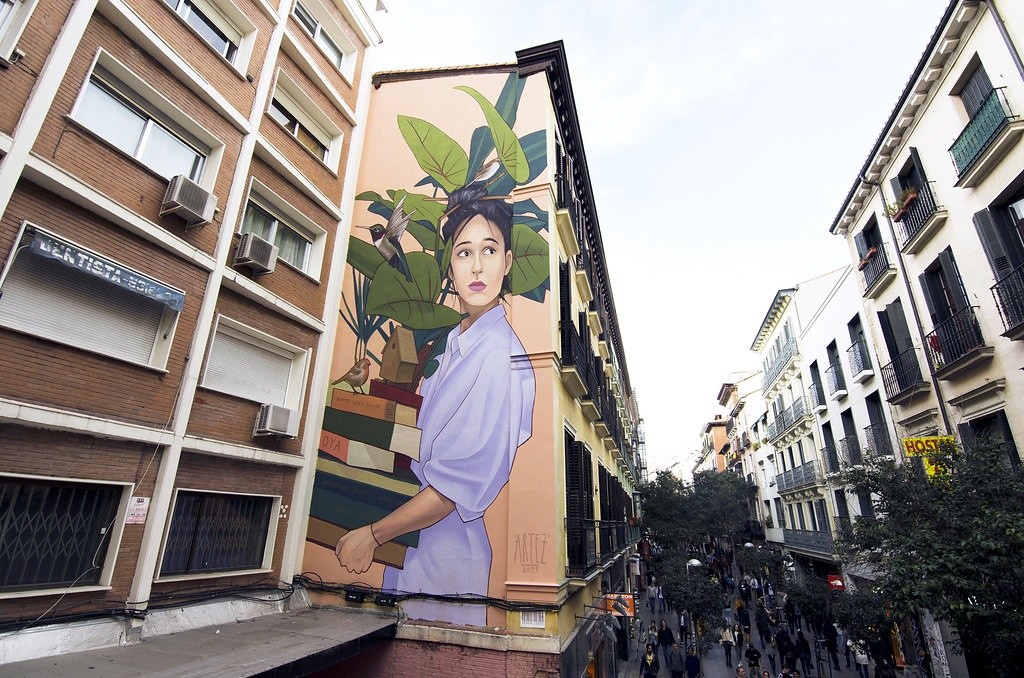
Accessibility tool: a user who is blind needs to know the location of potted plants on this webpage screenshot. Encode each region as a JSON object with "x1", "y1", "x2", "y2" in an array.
[{"x1": 882, "y1": 186, "x2": 918, "y2": 222}]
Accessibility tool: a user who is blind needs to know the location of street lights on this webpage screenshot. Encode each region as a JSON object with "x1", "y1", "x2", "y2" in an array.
[
  {"x1": 745, "y1": 542, "x2": 767, "y2": 608},
  {"x1": 685, "y1": 558, "x2": 703, "y2": 653}
]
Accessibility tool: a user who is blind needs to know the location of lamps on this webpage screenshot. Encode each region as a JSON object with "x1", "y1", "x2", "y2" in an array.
[{"x1": 575, "y1": 595, "x2": 629, "y2": 643}]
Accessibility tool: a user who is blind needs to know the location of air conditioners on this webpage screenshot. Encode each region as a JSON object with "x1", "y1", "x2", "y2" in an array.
[
  {"x1": 235, "y1": 233, "x2": 279, "y2": 273},
  {"x1": 256, "y1": 404, "x2": 302, "y2": 437},
  {"x1": 162, "y1": 174, "x2": 218, "y2": 225}
]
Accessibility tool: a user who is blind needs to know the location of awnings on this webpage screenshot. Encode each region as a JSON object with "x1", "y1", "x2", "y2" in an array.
[{"x1": 0, "y1": 220, "x2": 187, "y2": 340}]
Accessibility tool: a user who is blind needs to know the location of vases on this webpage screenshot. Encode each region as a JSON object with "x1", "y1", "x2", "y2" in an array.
[{"x1": 857, "y1": 247, "x2": 878, "y2": 271}]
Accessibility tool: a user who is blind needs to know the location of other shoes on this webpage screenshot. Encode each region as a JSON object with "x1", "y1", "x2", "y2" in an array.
[
  {"x1": 738, "y1": 663, "x2": 741, "y2": 667},
  {"x1": 846, "y1": 665, "x2": 851, "y2": 669},
  {"x1": 729, "y1": 664, "x2": 733, "y2": 668},
  {"x1": 740, "y1": 661, "x2": 742, "y2": 665},
  {"x1": 834, "y1": 666, "x2": 840, "y2": 671},
  {"x1": 726, "y1": 663, "x2": 729, "y2": 666}
]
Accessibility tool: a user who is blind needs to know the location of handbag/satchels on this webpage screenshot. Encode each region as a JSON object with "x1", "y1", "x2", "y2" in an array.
[{"x1": 810, "y1": 660, "x2": 814, "y2": 669}]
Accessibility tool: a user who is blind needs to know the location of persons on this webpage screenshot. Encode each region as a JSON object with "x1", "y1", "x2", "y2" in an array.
[{"x1": 604, "y1": 530, "x2": 908, "y2": 678}]
